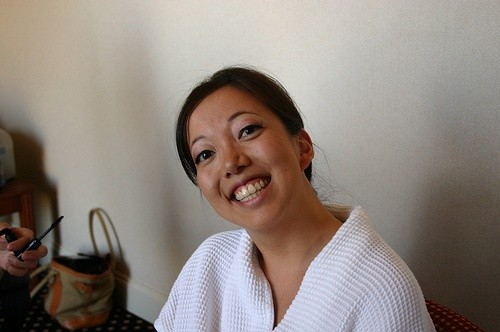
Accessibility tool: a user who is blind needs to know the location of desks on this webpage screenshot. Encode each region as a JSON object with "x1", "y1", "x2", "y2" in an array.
[{"x1": 0, "y1": 179, "x2": 39, "y2": 294}]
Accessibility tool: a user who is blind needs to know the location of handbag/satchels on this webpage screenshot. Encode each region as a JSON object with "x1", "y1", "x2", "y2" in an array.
[{"x1": 28, "y1": 209, "x2": 115, "y2": 330}]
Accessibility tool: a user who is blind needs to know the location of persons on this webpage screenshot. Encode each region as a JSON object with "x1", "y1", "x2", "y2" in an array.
[
  {"x1": 152, "y1": 67, "x2": 436, "y2": 332},
  {"x1": 0, "y1": 222, "x2": 47, "y2": 278}
]
